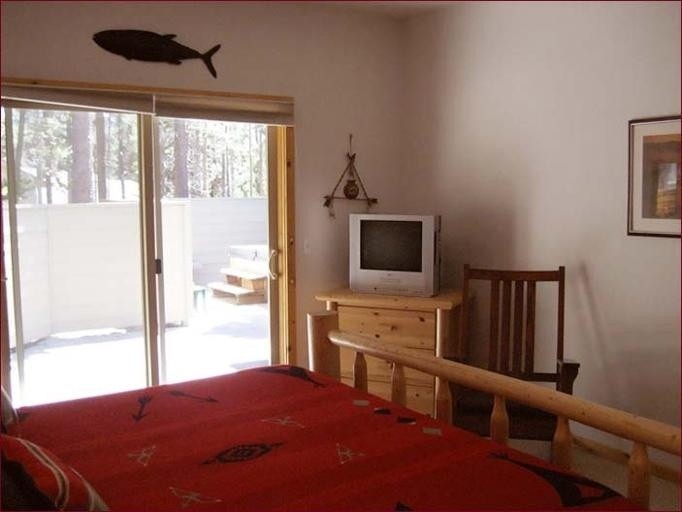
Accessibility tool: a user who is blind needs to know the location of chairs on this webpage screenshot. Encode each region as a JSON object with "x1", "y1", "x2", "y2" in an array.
[{"x1": 441, "y1": 262, "x2": 580, "y2": 441}]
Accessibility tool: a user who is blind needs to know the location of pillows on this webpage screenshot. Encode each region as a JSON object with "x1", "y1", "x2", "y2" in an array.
[
  {"x1": 0, "y1": 385, "x2": 21, "y2": 439},
  {"x1": 0, "y1": 434, "x2": 109, "y2": 512}
]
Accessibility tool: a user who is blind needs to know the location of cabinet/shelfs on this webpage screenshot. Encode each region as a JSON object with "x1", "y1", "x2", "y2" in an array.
[{"x1": 315, "y1": 281, "x2": 478, "y2": 425}]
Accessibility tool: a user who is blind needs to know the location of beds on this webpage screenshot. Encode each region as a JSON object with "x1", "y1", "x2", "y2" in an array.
[{"x1": 0, "y1": 311, "x2": 682, "y2": 511}]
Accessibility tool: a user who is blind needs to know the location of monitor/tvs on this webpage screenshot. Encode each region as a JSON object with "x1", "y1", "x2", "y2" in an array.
[{"x1": 348, "y1": 214, "x2": 442, "y2": 297}]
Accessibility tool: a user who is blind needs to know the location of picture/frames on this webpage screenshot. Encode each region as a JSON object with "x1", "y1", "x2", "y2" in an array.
[{"x1": 627, "y1": 115, "x2": 682, "y2": 240}]
